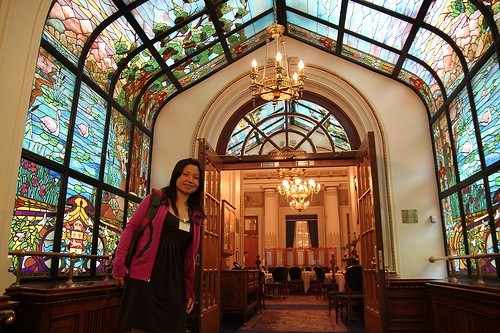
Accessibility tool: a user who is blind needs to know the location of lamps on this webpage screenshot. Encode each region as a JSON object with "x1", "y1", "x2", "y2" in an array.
[
  {"x1": 276, "y1": 168, "x2": 322, "y2": 212},
  {"x1": 249, "y1": 0, "x2": 307, "y2": 110}
]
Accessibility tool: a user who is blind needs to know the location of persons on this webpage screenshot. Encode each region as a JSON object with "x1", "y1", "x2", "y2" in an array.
[
  {"x1": 111, "y1": 158, "x2": 206, "y2": 333},
  {"x1": 244, "y1": 251, "x2": 249, "y2": 257},
  {"x1": 345, "y1": 258, "x2": 363, "y2": 291}
]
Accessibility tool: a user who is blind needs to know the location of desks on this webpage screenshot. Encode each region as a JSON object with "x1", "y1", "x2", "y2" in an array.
[{"x1": 264, "y1": 273, "x2": 345, "y2": 293}]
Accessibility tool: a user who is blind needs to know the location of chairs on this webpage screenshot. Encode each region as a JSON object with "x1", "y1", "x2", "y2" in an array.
[{"x1": 256, "y1": 265, "x2": 364, "y2": 325}]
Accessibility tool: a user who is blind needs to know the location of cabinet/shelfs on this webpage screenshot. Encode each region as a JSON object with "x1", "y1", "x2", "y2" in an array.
[
  {"x1": 221, "y1": 270, "x2": 263, "y2": 327},
  {"x1": 8, "y1": 280, "x2": 123, "y2": 333}
]
